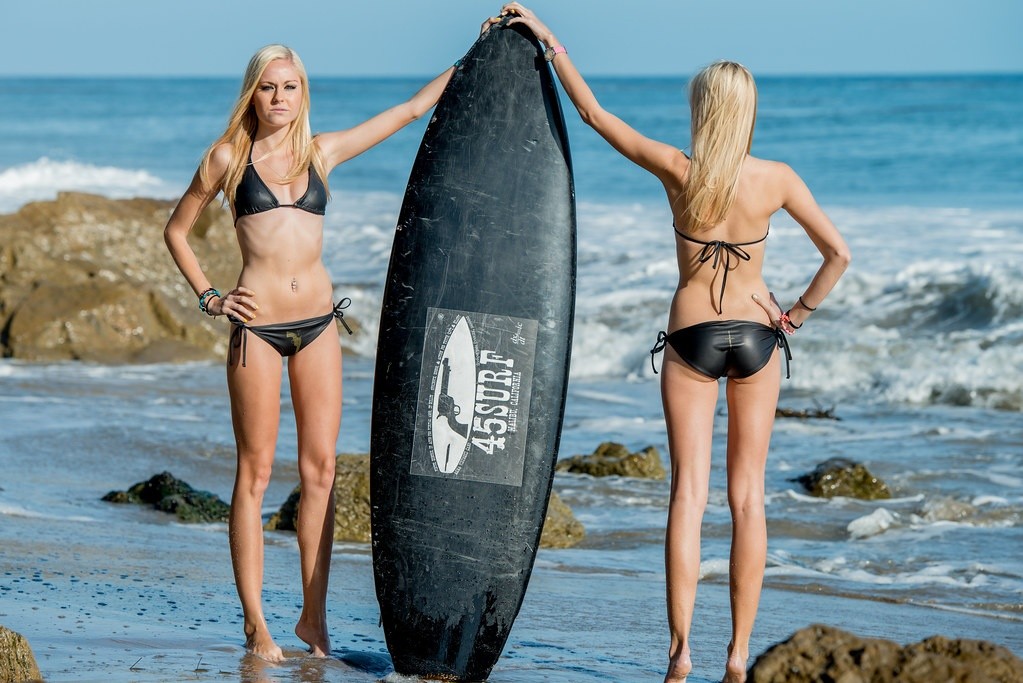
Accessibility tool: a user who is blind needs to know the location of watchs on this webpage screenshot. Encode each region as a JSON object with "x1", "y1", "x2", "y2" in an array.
[{"x1": 543, "y1": 45, "x2": 567, "y2": 62}]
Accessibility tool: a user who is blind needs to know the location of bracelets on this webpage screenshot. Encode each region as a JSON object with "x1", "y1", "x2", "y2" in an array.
[
  {"x1": 799, "y1": 296, "x2": 816, "y2": 311},
  {"x1": 198, "y1": 287, "x2": 220, "y2": 316},
  {"x1": 454, "y1": 59, "x2": 462, "y2": 68},
  {"x1": 780, "y1": 309, "x2": 803, "y2": 335}
]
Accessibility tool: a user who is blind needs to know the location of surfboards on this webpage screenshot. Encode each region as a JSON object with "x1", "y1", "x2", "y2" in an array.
[{"x1": 367, "y1": 13, "x2": 579, "y2": 683}]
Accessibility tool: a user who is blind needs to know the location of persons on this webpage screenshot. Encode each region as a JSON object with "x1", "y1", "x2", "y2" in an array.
[
  {"x1": 501, "y1": 2, "x2": 851, "y2": 683},
  {"x1": 164, "y1": 14, "x2": 506, "y2": 661}
]
